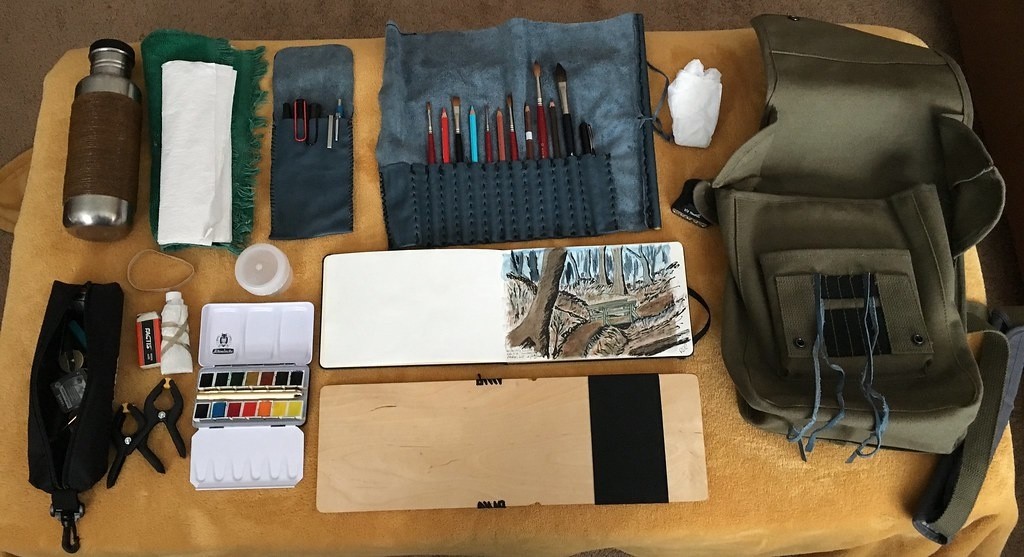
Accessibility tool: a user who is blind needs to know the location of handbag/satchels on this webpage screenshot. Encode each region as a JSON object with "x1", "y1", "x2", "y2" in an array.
[
  {"x1": 693, "y1": 14, "x2": 1022, "y2": 546},
  {"x1": 29, "y1": 280, "x2": 126, "y2": 553}
]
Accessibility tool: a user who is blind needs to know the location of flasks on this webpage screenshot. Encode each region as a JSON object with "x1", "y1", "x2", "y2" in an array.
[{"x1": 60, "y1": 38, "x2": 144, "y2": 243}]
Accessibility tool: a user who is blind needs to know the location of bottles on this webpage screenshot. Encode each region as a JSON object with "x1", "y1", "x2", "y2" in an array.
[{"x1": 161, "y1": 291, "x2": 193, "y2": 374}]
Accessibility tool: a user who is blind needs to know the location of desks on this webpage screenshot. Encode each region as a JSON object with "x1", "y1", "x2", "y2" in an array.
[{"x1": 0, "y1": 25, "x2": 1019, "y2": 557}]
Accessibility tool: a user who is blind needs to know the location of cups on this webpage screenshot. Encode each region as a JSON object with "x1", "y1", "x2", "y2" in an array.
[{"x1": 235, "y1": 243, "x2": 293, "y2": 296}]
